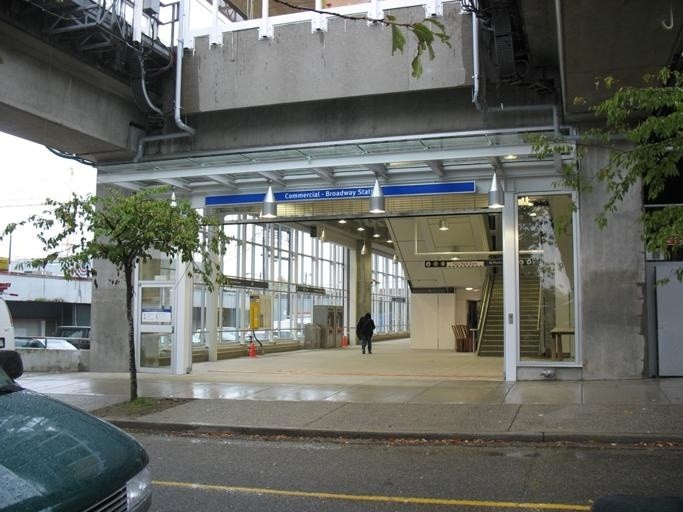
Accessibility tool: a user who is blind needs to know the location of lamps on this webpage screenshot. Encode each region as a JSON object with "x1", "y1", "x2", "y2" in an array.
[
  {"x1": 261, "y1": 184, "x2": 277, "y2": 219},
  {"x1": 486, "y1": 166, "x2": 506, "y2": 210},
  {"x1": 368, "y1": 176, "x2": 384, "y2": 215}
]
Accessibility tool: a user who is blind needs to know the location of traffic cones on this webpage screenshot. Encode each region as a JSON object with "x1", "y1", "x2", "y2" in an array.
[
  {"x1": 248, "y1": 343, "x2": 256, "y2": 356},
  {"x1": 343, "y1": 336, "x2": 347, "y2": 346}
]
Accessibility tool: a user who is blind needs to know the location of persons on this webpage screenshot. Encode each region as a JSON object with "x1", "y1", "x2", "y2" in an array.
[{"x1": 356, "y1": 313, "x2": 375, "y2": 354}]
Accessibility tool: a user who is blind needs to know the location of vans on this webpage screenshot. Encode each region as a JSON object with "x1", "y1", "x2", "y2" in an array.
[{"x1": 56, "y1": 326, "x2": 90, "y2": 348}]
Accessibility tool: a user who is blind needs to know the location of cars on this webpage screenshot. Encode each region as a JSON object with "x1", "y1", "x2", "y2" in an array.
[
  {"x1": 1, "y1": 367, "x2": 152, "y2": 512},
  {"x1": 24, "y1": 337, "x2": 77, "y2": 350}
]
